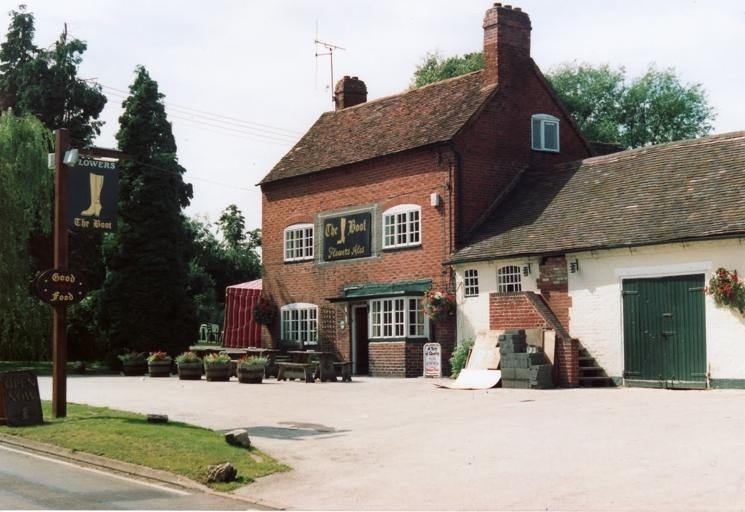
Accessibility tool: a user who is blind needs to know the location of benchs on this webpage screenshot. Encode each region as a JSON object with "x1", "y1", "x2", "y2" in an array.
[
  {"x1": 314, "y1": 361, "x2": 351, "y2": 382},
  {"x1": 275, "y1": 361, "x2": 313, "y2": 382}
]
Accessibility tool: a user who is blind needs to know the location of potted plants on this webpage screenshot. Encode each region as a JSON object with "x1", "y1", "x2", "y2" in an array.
[
  {"x1": 145, "y1": 351, "x2": 172, "y2": 377},
  {"x1": 117, "y1": 350, "x2": 145, "y2": 376},
  {"x1": 235, "y1": 354, "x2": 269, "y2": 383},
  {"x1": 204, "y1": 352, "x2": 233, "y2": 382},
  {"x1": 174, "y1": 351, "x2": 203, "y2": 379}
]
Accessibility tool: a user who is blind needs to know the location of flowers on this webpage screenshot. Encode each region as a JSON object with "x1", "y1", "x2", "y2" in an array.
[
  {"x1": 419, "y1": 287, "x2": 458, "y2": 323},
  {"x1": 709, "y1": 266, "x2": 744, "y2": 315}
]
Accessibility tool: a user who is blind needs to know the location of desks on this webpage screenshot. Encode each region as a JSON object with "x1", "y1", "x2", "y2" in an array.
[{"x1": 285, "y1": 350, "x2": 337, "y2": 382}]
